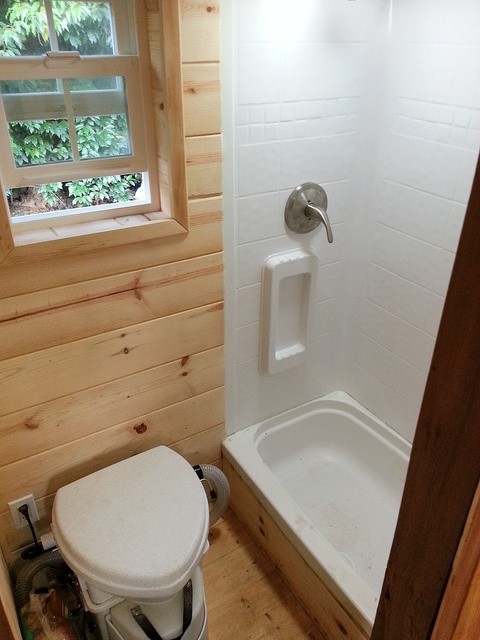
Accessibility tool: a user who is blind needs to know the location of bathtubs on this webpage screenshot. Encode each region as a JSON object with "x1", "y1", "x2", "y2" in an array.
[{"x1": 252, "y1": 399, "x2": 412, "y2": 605}]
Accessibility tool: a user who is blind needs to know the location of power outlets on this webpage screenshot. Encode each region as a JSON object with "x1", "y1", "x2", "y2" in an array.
[{"x1": 7, "y1": 492, "x2": 40, "y2": 530}]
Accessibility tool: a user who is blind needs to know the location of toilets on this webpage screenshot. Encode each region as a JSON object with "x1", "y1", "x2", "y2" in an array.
[{"x1": 50, "y1": 444, "x2": 211, "y2": 640}]
